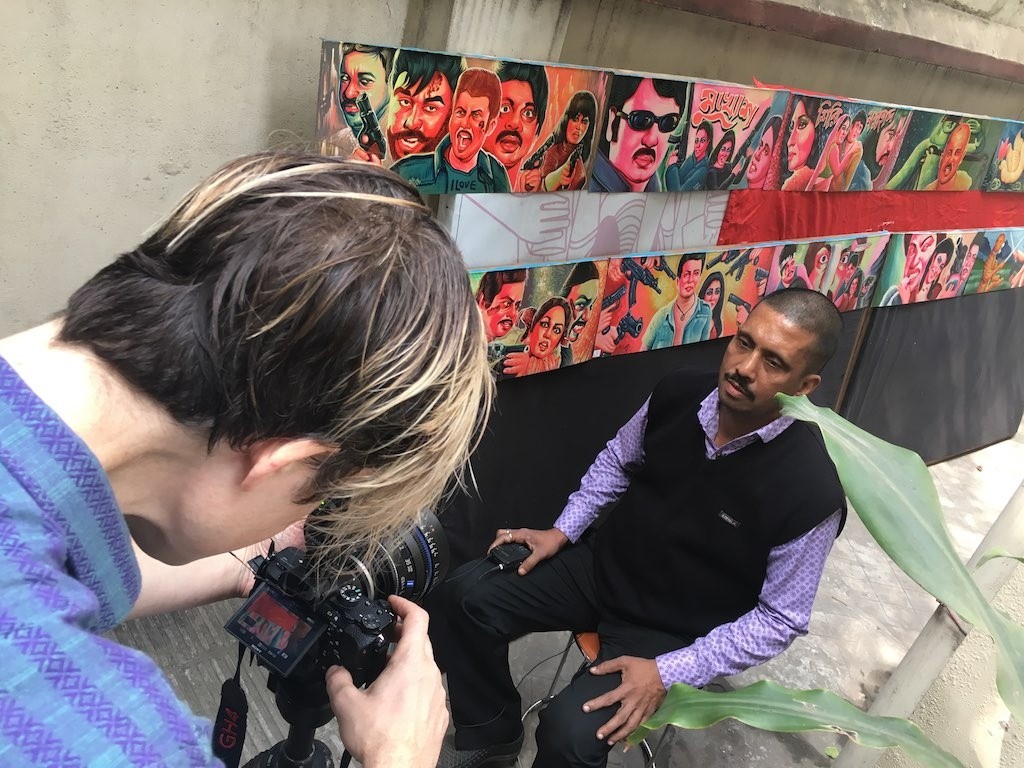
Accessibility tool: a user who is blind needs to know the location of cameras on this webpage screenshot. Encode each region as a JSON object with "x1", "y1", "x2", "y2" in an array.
[{"x1": 224, "y1": 508, "x2": 454, "y2": 709}]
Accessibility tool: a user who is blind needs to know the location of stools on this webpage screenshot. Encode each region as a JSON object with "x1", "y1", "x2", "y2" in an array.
[{"x1": 519, "y1": 631, "x2": 674, "y2": 768}]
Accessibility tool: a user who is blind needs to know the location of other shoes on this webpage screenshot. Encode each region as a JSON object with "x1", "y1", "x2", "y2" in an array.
[{"x1": 436, "y1": 721, "x2": 524, "y2": 768}]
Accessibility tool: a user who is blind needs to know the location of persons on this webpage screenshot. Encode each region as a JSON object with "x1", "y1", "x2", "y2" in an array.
[
  {"x1": 258, "y1": 620, "x2": 268, "y2": 639},
  {"x1": 0, "y1": 138, "x2": 497, "y2": 768},
  {"x1": 448, "y1": 287, "x2": 848, "y2": 768},
  {"x1": 276, "y1": 630, "x2": 290, "y2": 650}
]
